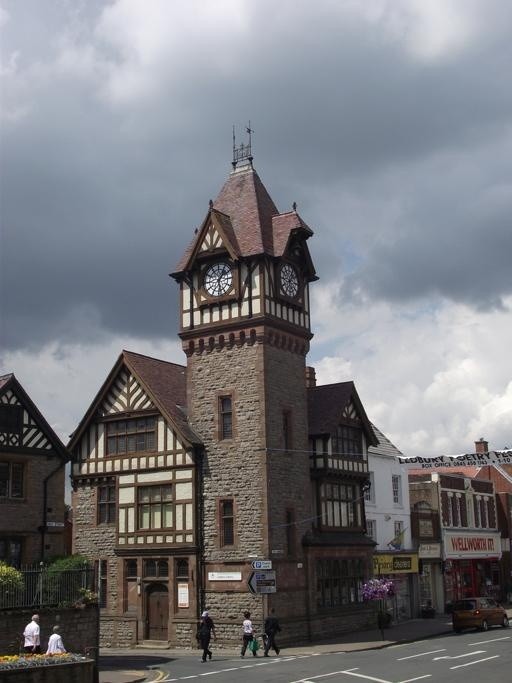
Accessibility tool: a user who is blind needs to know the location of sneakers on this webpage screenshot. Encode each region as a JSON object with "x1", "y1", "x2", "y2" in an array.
[
  {"x1": 200, "y1": 651, "x2": 213, "y2": 663},
  {"x1": 239, "y1": 649, "x2": 283, "y2": 658}
]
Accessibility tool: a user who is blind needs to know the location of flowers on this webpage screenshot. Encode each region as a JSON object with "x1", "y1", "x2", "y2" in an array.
[{"x1": 361, "y1": 577, "x2": 399, "y2": 601}]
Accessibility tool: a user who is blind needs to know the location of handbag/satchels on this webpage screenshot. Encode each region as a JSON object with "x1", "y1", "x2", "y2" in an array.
[
  {"x1": 248, "y1": 635, "x2": 259, "y2": 652},
  {"x1": 195, "y1": 630, "x2": 202, "y2": 640}
]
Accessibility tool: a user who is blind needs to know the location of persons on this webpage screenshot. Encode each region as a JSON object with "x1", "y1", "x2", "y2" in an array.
[
  {"x1": 263, "y1": 606, "x2": 282, "y2": 657},
  {"x1": 45, "y1": 624, "x2": 67, "y2": 654},
  {"x1": 239, "y1": 610, "x2": 258, "y2": 658},
  {"x1": 198, "y1": 610, "x2": 217, "y2": 662},
  {"x1": 21, "y1": 613, "x2": 41, "y2": 653}
]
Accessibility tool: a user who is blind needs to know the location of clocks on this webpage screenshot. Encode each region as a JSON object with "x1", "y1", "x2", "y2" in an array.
[
  {"x1": 279, "y1": 260, "x2": 304, "y2": 301},
  {"x1": 200, "y1": 257, "x2": 234, "y2": 299}
]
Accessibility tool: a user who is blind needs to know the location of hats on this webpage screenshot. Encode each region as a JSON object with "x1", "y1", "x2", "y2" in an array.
[{"x1": 202, "y1": 611, "x2": 209, "y2": 617}]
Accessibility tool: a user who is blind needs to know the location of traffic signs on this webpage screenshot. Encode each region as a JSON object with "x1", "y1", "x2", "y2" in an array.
[
  {"x1": 247, "y1": 571, "x2": 278, "y2": 595},
  {"x1": 251, "y1": 560, "x2": 274, "y2": 570}
]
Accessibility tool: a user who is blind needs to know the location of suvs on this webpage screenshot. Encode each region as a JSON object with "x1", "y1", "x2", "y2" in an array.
[{"x1": 453, "y1": 597, "x2": 510, "y2": 635}]
